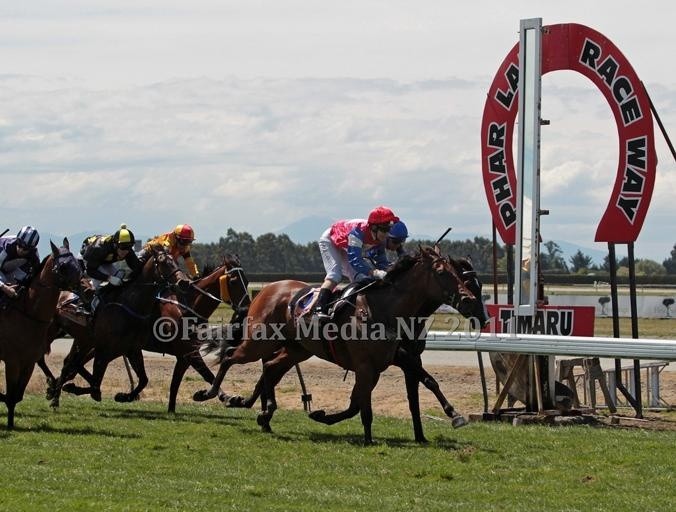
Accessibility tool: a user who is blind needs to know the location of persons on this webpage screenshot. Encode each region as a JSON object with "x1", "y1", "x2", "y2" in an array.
[
  {"x1": 136, "y1": 223, "x2": 199, "y2": 289},
  {"x1": 75, "y1": 223, "x2": 144, "y2": 317},
  {"x1": 308, "y1": 204, "x2": 400, "y2": 320},
  {"x1": 0, "y1": 223, "x2": 43, "y2": 302},
  {"x1": 363, "y1": 218, "x2": 409, "y2": 272}
]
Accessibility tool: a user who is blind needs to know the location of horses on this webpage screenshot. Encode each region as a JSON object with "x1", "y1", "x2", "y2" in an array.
[
  {"x1": 257, "y1": 251, "x2": 490, "y2": 446},
  {"x1": 50, "y1": 252, "x2": 251, "y2": 407},
  {"x1": 0, "y1": 237, "x2": 97, "y2": 428},
  {"x1": 38, "y1": 243, "x2": 190, "y2": 405},
  {"x1": 187, "y1": 242, "x2": 477, "y2": 447}
]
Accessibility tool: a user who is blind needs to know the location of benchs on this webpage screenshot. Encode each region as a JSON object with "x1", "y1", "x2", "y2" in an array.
[{"x1": 601, "y1": 356, "x2": 667, "y2": 408}]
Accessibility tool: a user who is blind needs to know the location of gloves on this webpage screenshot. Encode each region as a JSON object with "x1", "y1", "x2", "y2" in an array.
[
  {"x1": 373, "y1": 268, "x2": 387, "y2": 280},
  {"x1": 108, "y1": 275, "x2": 122, "y2": 286}
]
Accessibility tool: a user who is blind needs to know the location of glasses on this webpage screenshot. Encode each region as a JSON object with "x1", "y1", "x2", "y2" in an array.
[
  {"x1": 118, "y1": 245, "x2": 132, "y2": 251},
  {"x1": 17, "y1": 243, "x2": 29, "y2": 251},
  {"x1": 390, "y1": 238, "x2": 403, "y2": 244},
  {"x1": 376, "y1": 226, "x2": 391, "y2": 233},
  {"x1": 175, "y1": 237, "x2": 192, "y2": 246}
]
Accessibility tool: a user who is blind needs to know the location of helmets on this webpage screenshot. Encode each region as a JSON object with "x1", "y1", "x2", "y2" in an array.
[
  {"x1": 17, "y1": 225, "x2": 40, "y2": 248},
  {"x1": 368, "y1": 206, "x2": 409, "y2": 242},
  {"x1": 113, "y1": 226, "x2": 136, "y2": 244},
  {"x1": 175, "y1": 224, "x2": 196, "y2": 241}
]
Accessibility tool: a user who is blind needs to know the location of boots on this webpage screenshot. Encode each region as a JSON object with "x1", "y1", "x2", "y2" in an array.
[{"x1": 309, "y1": 287, "x2": 334, "y2": 320}]
